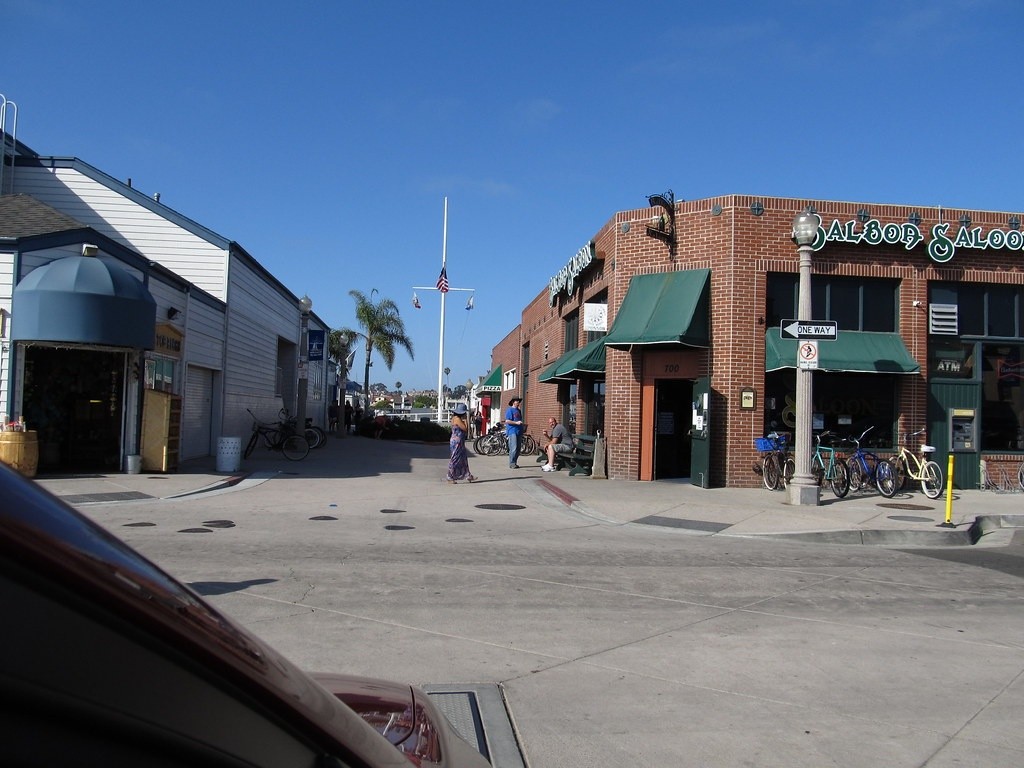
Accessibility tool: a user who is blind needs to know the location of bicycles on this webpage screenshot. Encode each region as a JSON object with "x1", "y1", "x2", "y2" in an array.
[
  {"x1": 762, "y1": 426, "x2": 945, "y2": 500},
  {"x1": 473, "y1": 420, "x2": 535, "y2": 456},
  {"x1": 243, "y1": 408, "x2": 327, "y2": 460}
]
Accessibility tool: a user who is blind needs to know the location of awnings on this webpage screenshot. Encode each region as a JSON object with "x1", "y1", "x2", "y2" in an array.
[
  {"x1": 604, "y1": 268, "x2": 711, "y2": 352},
  {"x1": 10, "y1": 254, "x2": 156, "y2": 349},
  {"x1": 765, "y1": 326, "x2": 920, "y2": 374},
  {"x1": 555, "y1": 336, "x2": 606, "y2": 376},
  {"x1": 475, "y1": 364, "x2": 502, "y2": 397},
  {"x1": 538, "y1": 349, "x2": 578, "y2": 384}
]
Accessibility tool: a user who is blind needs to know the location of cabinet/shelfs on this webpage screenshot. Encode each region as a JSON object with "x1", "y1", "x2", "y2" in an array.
[{"x1": 140, "y1": 390, "x2": 182, "y2": 473}]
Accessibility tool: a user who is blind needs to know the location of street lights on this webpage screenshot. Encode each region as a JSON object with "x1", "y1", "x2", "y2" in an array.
[
  {"x1": 336, "y1": 332, "x2": 348, "y2": 439},
  {"x1": 787, "y1": 208, "x2": 821, "y2": 505},
  {"x1": 466, "y1": 379, "x2": 474, "y2": 440},
  {"x1": 290, "y1": 294, "x2": 309, "y2": 453}
]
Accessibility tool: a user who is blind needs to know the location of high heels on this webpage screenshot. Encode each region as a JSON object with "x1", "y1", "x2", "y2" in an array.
[
  {"x1": 467, "y1": 476, "x2": 479, "y2": 482},
  {"x1": 447, "y1": 479, "x2": 457, "y2": 484}
]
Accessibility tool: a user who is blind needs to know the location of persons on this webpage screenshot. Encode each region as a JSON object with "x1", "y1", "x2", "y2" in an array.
[
  {"x1": 447, "y1": 403, "x2": 478, "y2": 484},
  {"x1": 475, "y1": 411, "x2": 482, "y2": 438},
  {"x1": 328, "y1": 399, "x2": 339, "y2": 432},
  {"x1": 354, "y1": 403, "x2": 362, "y2": 431},
  {"x1": 344, "y1": 400, "x2": 354, "y2": 435},
  {"x1": 505, "y1": 395, "x2": 523, "y2": 468},
  {"x1": 540, "y1": 417, "x2": 575, "y2": 472}
]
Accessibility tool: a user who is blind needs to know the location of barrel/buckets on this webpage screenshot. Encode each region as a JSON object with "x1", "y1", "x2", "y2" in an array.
[
  {"x1": 123, "y1": 454, "x2": 143, "y2": 474},
  {"x1": 0, "y1": 429, "x2": 38, "y2": 478}
]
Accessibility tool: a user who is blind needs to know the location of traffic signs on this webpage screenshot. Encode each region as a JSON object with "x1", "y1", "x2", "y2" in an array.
[{"x1": 778, "y1": 318, "x2": 838, "y2": 341}]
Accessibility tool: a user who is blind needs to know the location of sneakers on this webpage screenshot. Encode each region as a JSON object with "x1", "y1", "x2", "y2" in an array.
[{"x1": 541, "y1": 463, "x2": 556, "y2": 472}]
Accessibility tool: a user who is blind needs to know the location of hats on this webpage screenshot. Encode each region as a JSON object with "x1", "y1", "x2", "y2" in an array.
[
  {"x1": 508, "y1": 395, "x2": 522, "y2": 406},
  {"x1": 449, "y1": 403, "x2": 470, "y2": 414}
]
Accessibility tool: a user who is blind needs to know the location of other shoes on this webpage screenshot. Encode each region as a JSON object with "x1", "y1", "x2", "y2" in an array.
[{"x1": 509, "y1": 464, "x2": 519, "y2": 469}]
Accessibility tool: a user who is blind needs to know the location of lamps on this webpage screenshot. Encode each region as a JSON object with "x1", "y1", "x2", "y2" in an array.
[
  {"x1": 167, "y1": 306, "x2": 182, "y2": 320},
  {"x1": 81, "y1": 244, "x2": 99, "y2": 257}
]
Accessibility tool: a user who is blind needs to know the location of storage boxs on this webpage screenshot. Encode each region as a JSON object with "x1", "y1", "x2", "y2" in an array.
[{"x1": 753, "y1": 437, "x2": 785, "y2": 452}]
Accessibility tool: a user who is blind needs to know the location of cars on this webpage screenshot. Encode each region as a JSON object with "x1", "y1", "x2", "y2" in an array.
[{"x1": 0, "y1": 462, "x2": 495, "y2": 768}]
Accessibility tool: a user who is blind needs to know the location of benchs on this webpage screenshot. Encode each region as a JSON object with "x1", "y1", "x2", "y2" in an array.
[{"x1": 536, "y1": 433, "x2": 597, "y2": 476}]
